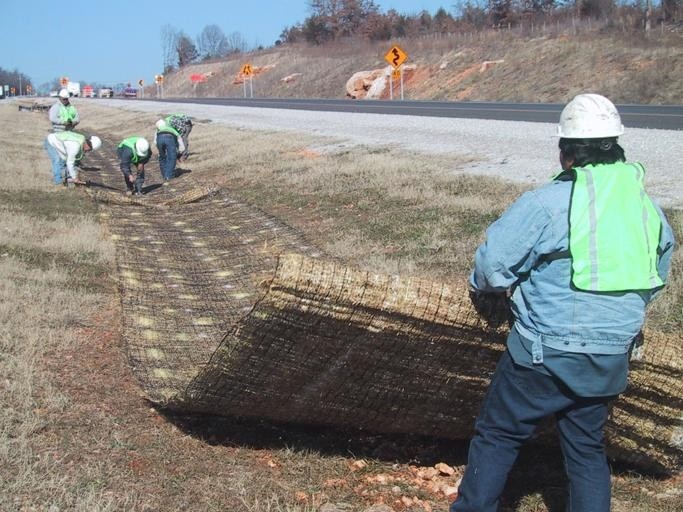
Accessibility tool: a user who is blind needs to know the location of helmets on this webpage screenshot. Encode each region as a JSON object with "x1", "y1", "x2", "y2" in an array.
[
  {"x1": 90, "y1": 135, "x2": 102, "y2": 152},
  {"x1": 59, "y1": 88, "x2": 70, "y2": 99},
  {"x1": 135, "y1": 138, "x2": 150, "y2": 157},
  {"x1": 155, "y1": 119, "x2": 166, "y2": 130},
  {"x1": 550, "y1": 93, "x2": 625, "y2": 141}
]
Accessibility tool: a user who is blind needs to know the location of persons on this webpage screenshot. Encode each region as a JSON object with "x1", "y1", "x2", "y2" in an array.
[
  {"x1": 453, "y1": 93, "x2": 675, "y2": 510},
  {"x1": 48, "y1": 89, "x2": 79, "y2": 131},
  {"x1": 154, "y1": 125, "x2": 185, "y2": 180},
  {"x1": 153, "y1": 114, "x2": 191, "y2": 160},
  {"x1": 116, "y1": 137, "x2": 150, "y2": 194},
  {"x1": 45, "y1": 132, "x2": 101, "y2": 186}
]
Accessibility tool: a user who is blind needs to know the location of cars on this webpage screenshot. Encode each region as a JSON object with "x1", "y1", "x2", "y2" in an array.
[{"x1": 82, "y1": 86, "x2": 137, "y2": 100}]
